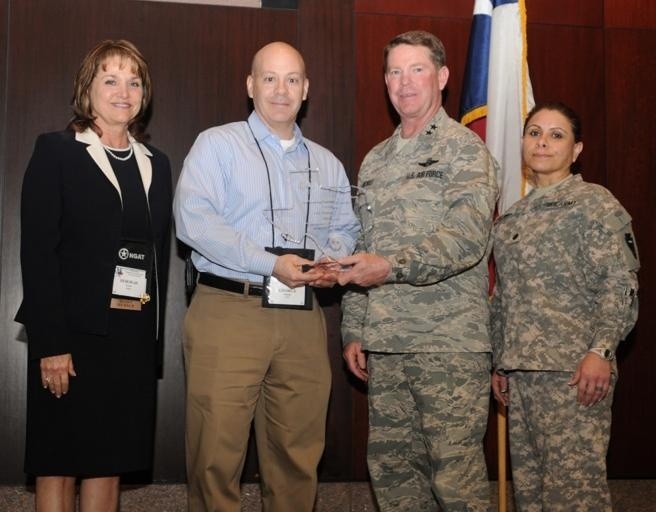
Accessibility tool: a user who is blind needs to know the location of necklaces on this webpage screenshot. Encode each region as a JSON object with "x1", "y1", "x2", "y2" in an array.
[{"x1": 100, "y1": 140, "x2": 134, "y2": 162}]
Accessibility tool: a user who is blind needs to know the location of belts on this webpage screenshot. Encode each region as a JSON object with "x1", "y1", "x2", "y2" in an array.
[{"x1": 195, "y1": 272, "x2": 267, "y2": 298}]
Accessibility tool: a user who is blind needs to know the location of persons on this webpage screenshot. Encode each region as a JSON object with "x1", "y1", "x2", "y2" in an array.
[
  {"x1": 12, "y1": 36, "x2": 173, "y2": 512},
  {"x1": 342, "y1": 29, "x2": 507, "y2": 512},
  {"x1": 487, "y1": 99, "x2": 641, "y2": 511},
  {"x1": 172, "y1": 40, "x2": 360, "y2": 511}
]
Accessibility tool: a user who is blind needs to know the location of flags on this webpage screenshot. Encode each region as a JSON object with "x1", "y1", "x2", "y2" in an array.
[{"x1": 458, "y1": 0, "x2": 538, "y2": 422}]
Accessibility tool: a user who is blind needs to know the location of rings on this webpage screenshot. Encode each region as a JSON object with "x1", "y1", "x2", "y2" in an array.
[
  {"x1": 596, "y1": 386, "x2": 603, "y2": 392},
  {"x1": 44, "y1": 377, "x2": 53, "y2": 384},
  {"x1": 603, "y1": 387, "x2": 608, "y2": 393}
]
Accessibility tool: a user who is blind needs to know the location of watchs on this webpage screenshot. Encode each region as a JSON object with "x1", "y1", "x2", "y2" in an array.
[{"x1": 589, "y1": 347, "x2": 613, "y2": 360}]
